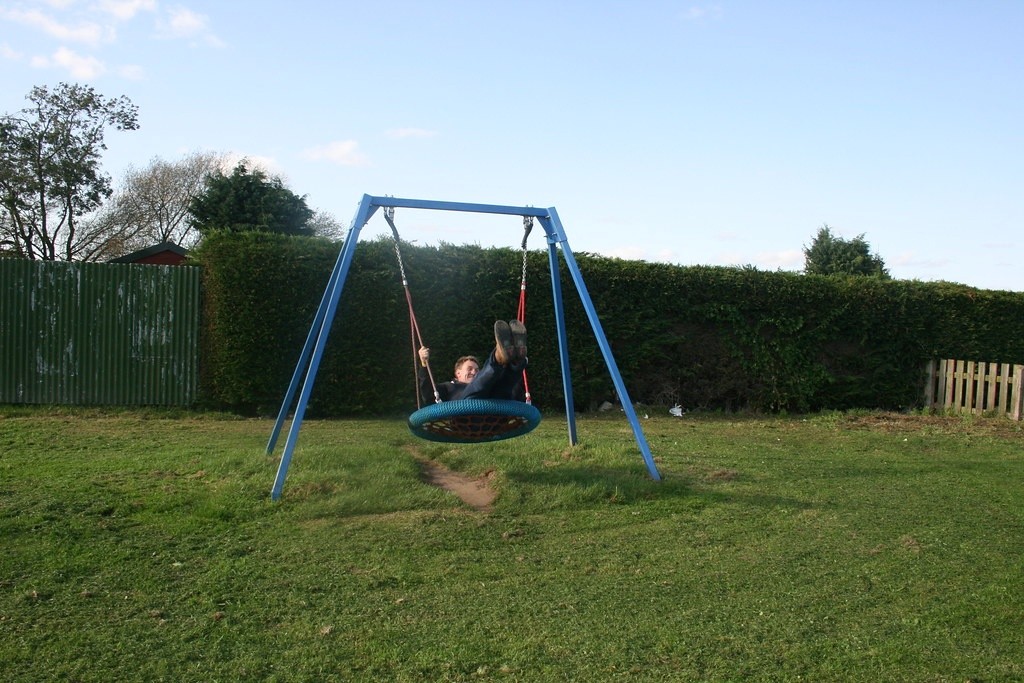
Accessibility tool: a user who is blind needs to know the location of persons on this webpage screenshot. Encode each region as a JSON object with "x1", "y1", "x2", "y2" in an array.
[{"x1": 418, "y1": 320, "x2": 529, "y2": 407}]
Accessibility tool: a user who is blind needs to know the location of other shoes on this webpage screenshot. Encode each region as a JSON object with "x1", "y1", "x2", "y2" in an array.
[{"x1": 494, "y1": 319, "x2": 529, "y2": 372}]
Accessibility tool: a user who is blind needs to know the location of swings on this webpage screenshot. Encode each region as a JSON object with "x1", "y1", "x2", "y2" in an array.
[{"x1": 382, "y1": 204, "x2": 544, "y2": 446}]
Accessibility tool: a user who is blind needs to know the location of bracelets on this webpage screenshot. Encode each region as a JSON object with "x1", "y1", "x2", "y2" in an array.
[{"x1": 422, "y1": 361, "x2": 427, "y2": 365}]
